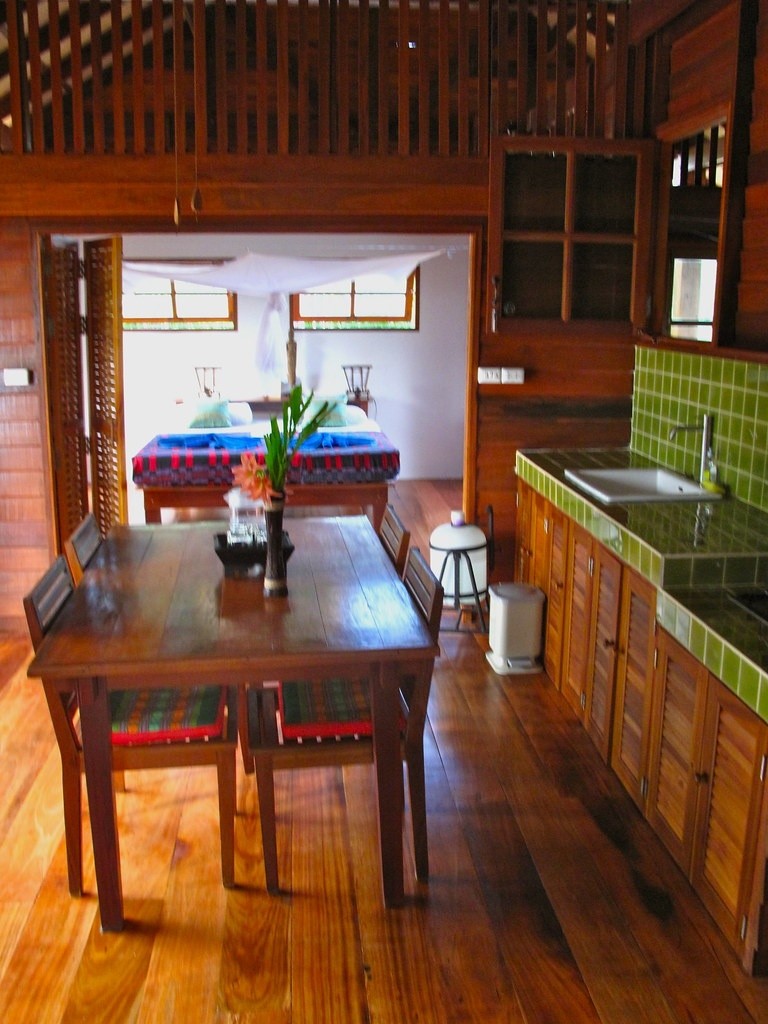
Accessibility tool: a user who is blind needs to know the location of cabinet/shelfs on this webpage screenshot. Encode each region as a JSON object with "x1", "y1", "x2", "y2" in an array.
[
  {"x1": 640, "y1": 618, "x2": 768, "y2": 980},
  {"x1": 486, "y1": 133, "x2": 659, "y2": 350},
  {"x1": 512, "y1": 476, "x2": 658, "y2": 816}
]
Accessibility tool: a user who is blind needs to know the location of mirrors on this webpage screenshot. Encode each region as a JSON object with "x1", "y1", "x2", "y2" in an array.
[{"x1": 653, "y1": 117, "x2": 727, "y2": 345}]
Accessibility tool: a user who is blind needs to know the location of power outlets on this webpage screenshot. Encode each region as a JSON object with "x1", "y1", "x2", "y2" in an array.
[{"x1": 478, "y1": 367, "x2": 524, "y2": 386}]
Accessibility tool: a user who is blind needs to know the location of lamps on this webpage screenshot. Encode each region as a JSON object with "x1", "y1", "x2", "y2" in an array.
[
  {"x1": 342, "y1": 364, "x2": 373, "y2": 401},
  {"x1": 194, "y1": 365, "x2": 221, "y2": 400}
]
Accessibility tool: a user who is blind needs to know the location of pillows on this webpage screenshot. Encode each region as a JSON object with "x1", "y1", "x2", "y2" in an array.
[
  {"x1": 186, "y1": 399, "x2": 232, "y2": 429},
  {"x1": 304, "y1": 393, "x2": 348, "y2": 427}
]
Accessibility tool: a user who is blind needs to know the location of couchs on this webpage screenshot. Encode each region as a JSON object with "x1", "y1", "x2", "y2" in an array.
[{"x1": 181, "y1": 401, "x2": 382, "y2": 432}]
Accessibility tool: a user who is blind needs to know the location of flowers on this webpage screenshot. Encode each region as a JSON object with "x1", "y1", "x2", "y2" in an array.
[{"x1": 232, "y1": 382, "x2": 336, "y2": 507}]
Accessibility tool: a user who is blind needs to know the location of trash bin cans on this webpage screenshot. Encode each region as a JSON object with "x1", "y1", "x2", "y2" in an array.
[{"x1": 483, "y1": 582, "x2": 548, "y2": 675}]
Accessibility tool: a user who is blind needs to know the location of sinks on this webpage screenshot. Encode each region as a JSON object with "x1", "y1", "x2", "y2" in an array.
[{"x1": 564, "y1": 466, "x2": 722, "y2": 507}]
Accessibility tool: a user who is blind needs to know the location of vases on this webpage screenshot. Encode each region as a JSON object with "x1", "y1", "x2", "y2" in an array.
[{"x1": 261, "y1": 491, "x2": 288, "y2": 598}]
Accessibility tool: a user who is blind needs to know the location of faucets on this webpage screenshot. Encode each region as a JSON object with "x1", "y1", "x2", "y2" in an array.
[{"x1": 669, "y1": 412, "x2": 712, "y2": 482}]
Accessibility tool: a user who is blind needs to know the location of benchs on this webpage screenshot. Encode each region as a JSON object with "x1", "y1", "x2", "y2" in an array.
[{"x1": 17, "y1": 504, "x2": 444, "y2": 895}]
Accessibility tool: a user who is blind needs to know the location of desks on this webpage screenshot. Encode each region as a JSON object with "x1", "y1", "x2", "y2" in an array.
[
  {"x1": 133, "y1": 436, "x2": 402, "y2": 523},
  {"x1": 27, "y1": 521, "x2": 440, "y2": 932},
  {"x1": 176, "y1": 398, "x2": 371, "y2": 422}
]
